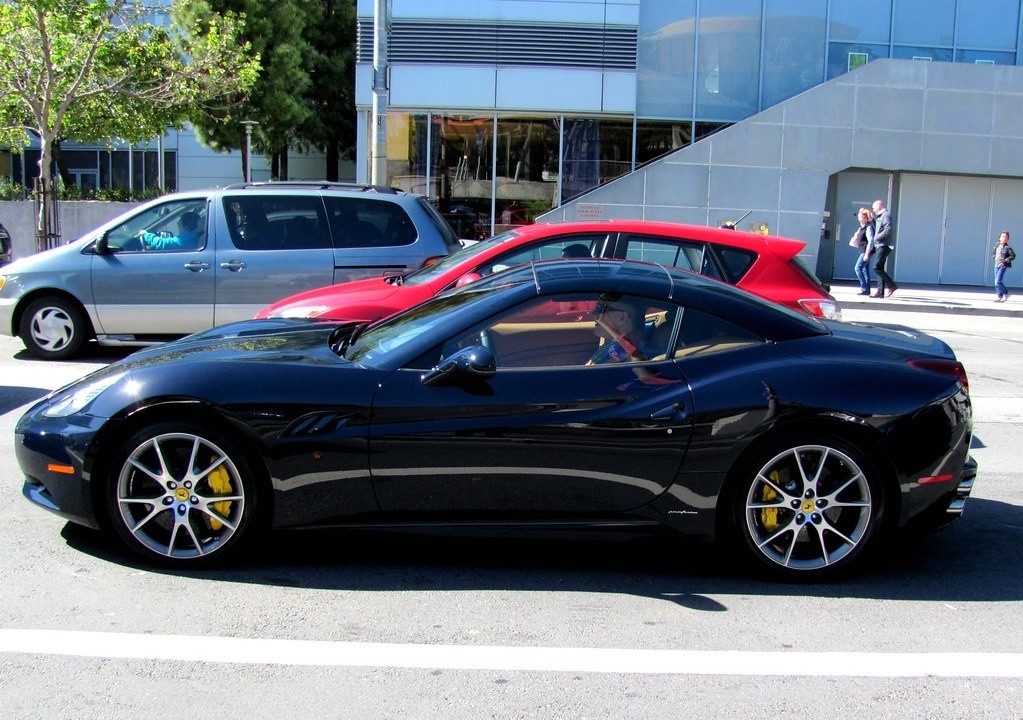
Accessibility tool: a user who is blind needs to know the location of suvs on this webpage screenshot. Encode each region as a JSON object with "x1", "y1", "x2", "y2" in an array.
[{"x1": 0, "y1": 179, "x2": 468, "y2": 361}]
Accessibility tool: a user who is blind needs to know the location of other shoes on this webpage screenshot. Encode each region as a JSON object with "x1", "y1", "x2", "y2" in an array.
[
  {"x1": 994, "y1": 297, "x2": 1002, "y2": 302},
  {"x1": 870, "y1": 292, "x2": 884, "y2": 298},
  {"x1": 858, "y1": 291, "x2": 870, "y2": 295},
  {"x1": 888, "y1": 287, "x2": 897, "y2": 297},
  {"x1": 1001, "y1": 292, "x2": 1011, "y2": 302}
]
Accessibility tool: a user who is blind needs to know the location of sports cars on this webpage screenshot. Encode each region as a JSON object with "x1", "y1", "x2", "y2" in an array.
[
  {"x1": 14, "y1": 257, "x2": 979, "y2": 584},
  {"x1": 254, "y1": 211, "x2": 841, "y2": 329}
]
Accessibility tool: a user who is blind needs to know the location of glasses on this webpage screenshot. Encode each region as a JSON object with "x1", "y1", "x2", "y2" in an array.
[{"x1": 595, "y1": 302, "x2": 631, "y2": 319}]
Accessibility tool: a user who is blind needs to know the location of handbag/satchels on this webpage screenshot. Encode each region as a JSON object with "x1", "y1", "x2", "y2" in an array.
[{"x1": 849, "y1": 227, "x2": 861, "y2": 248}]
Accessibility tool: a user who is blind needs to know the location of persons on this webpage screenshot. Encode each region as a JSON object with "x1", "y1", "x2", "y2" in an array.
[
  {"x1": 869, "y1": 199, "x2": 896, "y2": 298},
  {"x1": 992, "y1": 232, "x2": 1016, "y2": 303},
  {"x1": 561, "y1": 243, "x2": 592, "y2": 259},
  {"x1": 853, "y1": 207, "x2": 875, "y2": 295},
  {"x1": 138, "y1": 212, "x2": 201, "y2": 250},
  {"x1": 585, "y1": 293, "x2": 667, "y2": 365}
]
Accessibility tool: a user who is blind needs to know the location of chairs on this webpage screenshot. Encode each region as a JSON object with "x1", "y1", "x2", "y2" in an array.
[
  {"x1": 594, "y1": 300, "x2": 645, "y2": 347},
  {"x1": 650, "y1": 309, "x2": 687, "y2": 360}
]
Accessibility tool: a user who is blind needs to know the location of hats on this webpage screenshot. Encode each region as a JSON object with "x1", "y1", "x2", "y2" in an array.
[{"x1": 181, "y1": 212, "x2": 201, "y2": 230}]
